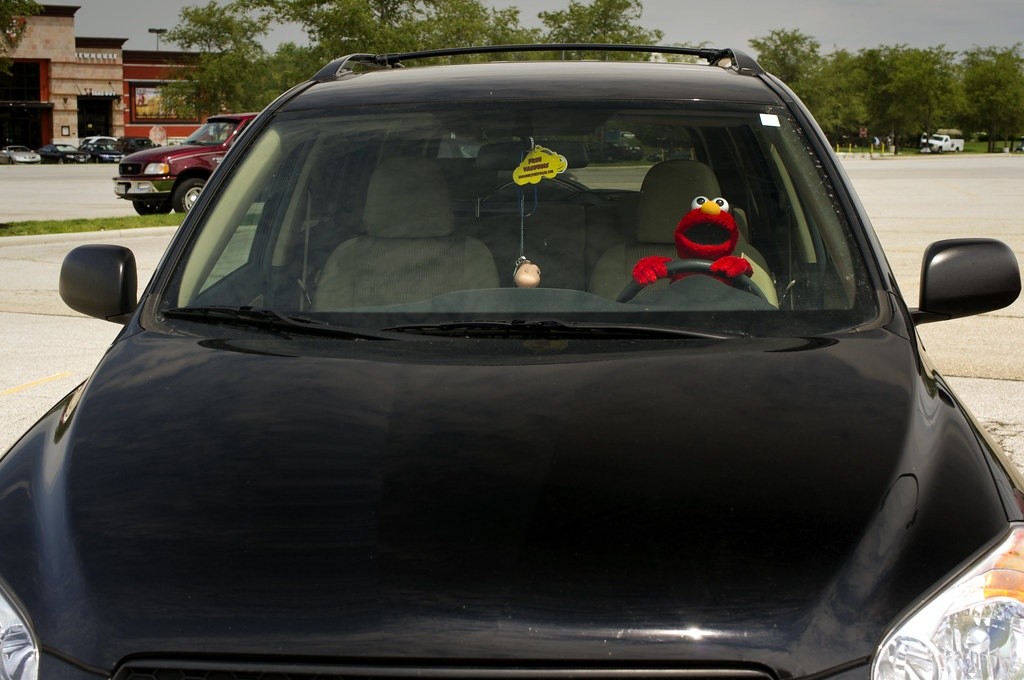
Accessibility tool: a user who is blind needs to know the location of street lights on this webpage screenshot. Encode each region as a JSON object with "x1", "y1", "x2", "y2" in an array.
[{"x1": 148, "y1": 28, "x2": 168, "y2": 51}]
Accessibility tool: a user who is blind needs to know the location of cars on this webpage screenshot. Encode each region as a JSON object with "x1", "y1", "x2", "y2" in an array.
[{"x1": 0, "y1": 136, "x2": 162, "y2": 165}]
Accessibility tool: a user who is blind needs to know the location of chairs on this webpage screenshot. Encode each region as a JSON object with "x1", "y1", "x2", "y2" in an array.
[{"x1": 315, "y1": 153, "x2": 778, "y2": 310}]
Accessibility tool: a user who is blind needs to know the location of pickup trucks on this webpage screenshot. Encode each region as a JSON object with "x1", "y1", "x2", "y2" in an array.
[{"x1": 920, "y1": 133, "x2": 964, "y2": 154}]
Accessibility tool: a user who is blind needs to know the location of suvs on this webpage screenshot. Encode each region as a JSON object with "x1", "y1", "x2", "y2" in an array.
[
  {"x1": 0, "y1": 43, "x2": 1024, "y2": 680},
  {"x1": 112, "y1": 112, "x2": 262, "y2": 216}
]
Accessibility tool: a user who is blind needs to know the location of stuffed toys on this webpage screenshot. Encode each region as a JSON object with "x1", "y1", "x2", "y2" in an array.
[{"x1": 628, "y1": 195, "x2": 753, "y2": 288}]
